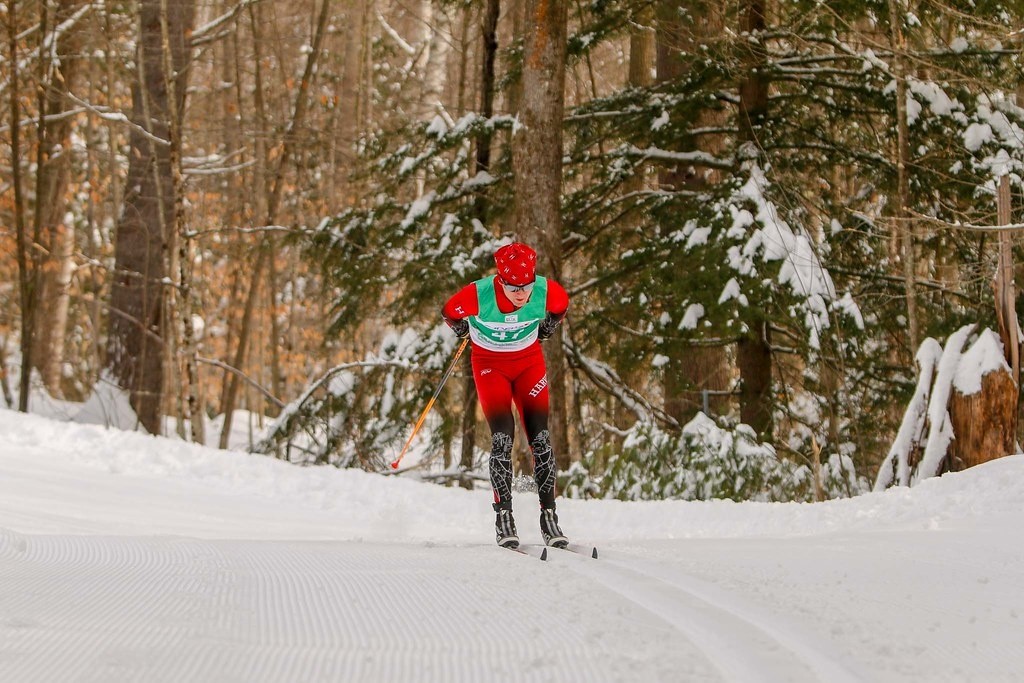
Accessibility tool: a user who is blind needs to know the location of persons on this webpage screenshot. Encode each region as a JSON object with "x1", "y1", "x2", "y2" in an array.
[{"x1": 442, "y1": 245, "x2": 569, "y2": 546}]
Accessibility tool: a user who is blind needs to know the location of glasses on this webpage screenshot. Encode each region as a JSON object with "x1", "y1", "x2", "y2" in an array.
[{"x1": 498, "y1": 270, "x2": 536, "y2": 293}]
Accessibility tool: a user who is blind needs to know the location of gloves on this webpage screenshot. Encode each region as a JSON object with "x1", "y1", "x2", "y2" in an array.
[
  {"x1": 538, "y1": 324, "x2": 553, "y2": 343},
  {"x1": 458, "y1": 326, "x2": 472, "y2": 352}
]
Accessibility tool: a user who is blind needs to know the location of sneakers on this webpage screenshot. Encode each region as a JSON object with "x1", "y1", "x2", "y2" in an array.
[
  {"x1": 539, "y1": 508, "x2": 570, "y2": 549},
  {"x1": 494, "y1": 509, "x2": 520, "y2": 548}
]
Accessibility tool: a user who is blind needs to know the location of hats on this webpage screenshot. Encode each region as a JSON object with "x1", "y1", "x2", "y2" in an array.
[{"x1": 493, "y1": 242, "x2": 537, "y2": 287}]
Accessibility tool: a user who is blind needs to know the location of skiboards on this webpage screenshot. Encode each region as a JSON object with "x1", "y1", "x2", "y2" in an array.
[
  {"x1": 555, "y1": 542, "x2": 598, "y2": 560},
  {"x1": 504, "y1": 542, "x2": 548, "y2": 561}
]
